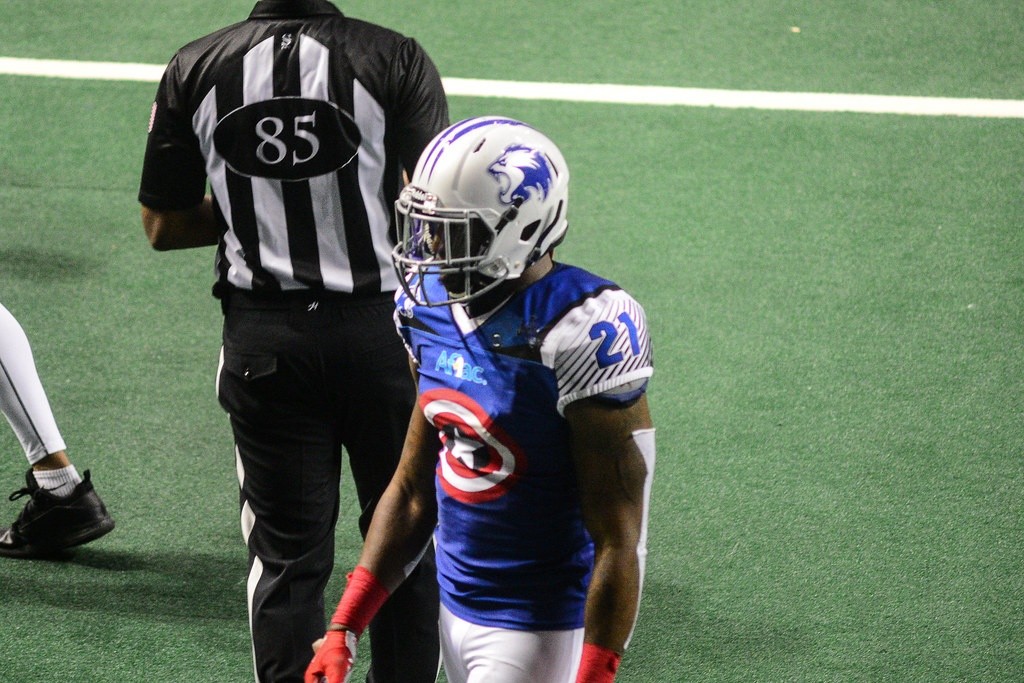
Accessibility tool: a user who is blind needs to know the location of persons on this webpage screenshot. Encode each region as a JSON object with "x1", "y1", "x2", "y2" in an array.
[
  {"x1": 138, "y1": 0, "x2": 450, "y2": 683},
  {"x1": 305, "y1": 116, "x2": 655, "y2": 683},
  {"x1": 0, "y1": 298, "x2": 115, "y2": 553}
]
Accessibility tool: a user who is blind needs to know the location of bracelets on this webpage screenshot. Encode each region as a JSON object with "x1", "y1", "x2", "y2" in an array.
[
  {"x1": 330, "y1": 566, "x2": 388, "y2": 634},
  {"x1": 575, "y1": 642, "x2": 622, "y2": 683}
]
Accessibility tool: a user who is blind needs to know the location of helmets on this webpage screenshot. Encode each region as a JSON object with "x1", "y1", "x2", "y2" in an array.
[{"x1": 394, "y1": 115, "x2": 569, "y2": 306}]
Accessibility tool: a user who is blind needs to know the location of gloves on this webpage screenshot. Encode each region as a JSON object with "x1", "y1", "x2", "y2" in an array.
[{"x1": 303, "y1": 629, "x2": 357, "y2": 683}]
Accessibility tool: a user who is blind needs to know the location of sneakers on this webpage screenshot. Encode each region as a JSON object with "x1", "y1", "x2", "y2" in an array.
[{"x1": 0, "y1": 467, "x2": 116, "y2": 556}]
